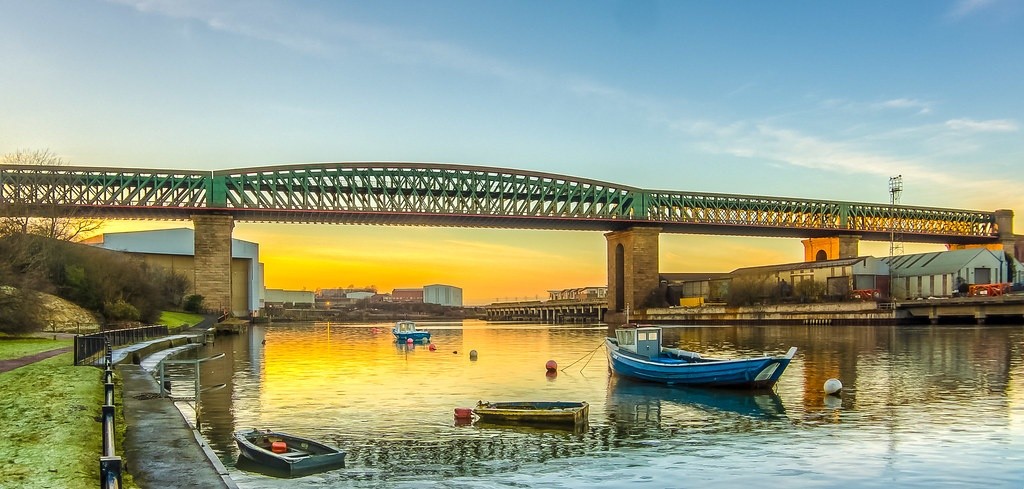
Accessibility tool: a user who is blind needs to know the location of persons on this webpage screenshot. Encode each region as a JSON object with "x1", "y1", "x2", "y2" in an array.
[{"x1": 224, "y1": 307, "x2": 228, "y2": 314}]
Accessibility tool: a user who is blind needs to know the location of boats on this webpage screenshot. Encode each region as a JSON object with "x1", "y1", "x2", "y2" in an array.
[
  {"x1": 604, "y1": 303, "x2": 799, "y2": 391},
  {"x1": 232, "y1": 427, "x2": 347, "y2": 471},
  {"x1": 391, "y1": 321, "x2": 431, "y2": 342},
  {"x1": 473, "y1": 400, "x2": 589, "y2": 425}
]
